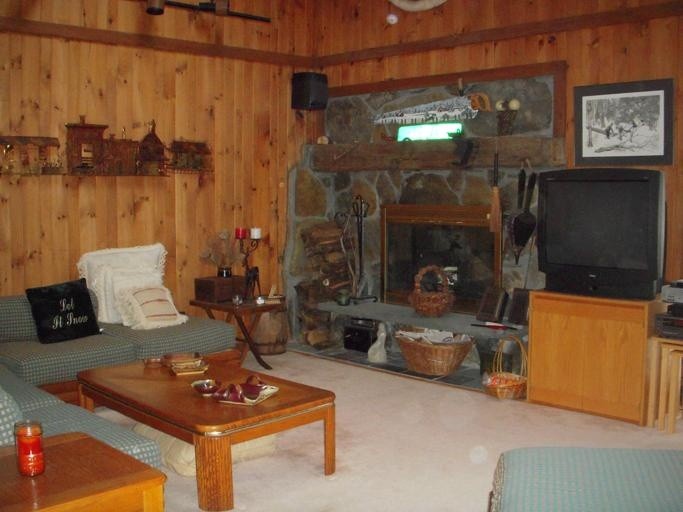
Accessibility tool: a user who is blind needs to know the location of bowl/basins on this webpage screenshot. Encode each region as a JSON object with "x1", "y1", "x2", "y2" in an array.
[{"x1": 189, "y1": 379, "x2": 221, "y2": 398}]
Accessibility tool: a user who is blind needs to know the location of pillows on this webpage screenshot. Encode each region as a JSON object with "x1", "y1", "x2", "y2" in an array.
[
  {"x1": 105, "y1": 265, "x2": 168, "y2": 327},
  {"x1": 75, "y1": 242, "x2": 167, "y2": 324},
  {"x1": 119, "y1": 285, "x2": 188, "y2": 331},
  {"x1": 25, "y1": 277, "x2": 101, "y2": 345}
]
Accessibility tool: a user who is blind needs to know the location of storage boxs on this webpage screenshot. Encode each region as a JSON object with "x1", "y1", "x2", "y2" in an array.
[{"x1": 194, "y1": 275, "x2": 245, "y2": 304}]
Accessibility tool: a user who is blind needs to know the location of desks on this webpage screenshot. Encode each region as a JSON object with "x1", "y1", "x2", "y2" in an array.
[
  {"x1": 189, "y1": 297, "x2": 288, "y2": 370},
  {"x1": 0, "y1": 429, "x2": 166, "y2": 511}
]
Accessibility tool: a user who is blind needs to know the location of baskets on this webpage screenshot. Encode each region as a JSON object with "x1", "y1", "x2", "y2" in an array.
[
  {"x1": 413, "y1": 265, "x2": 452, "y2": 318},
  {"x1": 397, "y1": 334, "x2": 471, "y2": 376},
  {"x1": 484, "y1": 335, "x2": 527, "y2": 399}
]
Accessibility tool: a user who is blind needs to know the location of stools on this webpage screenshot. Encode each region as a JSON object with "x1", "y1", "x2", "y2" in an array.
[
  {"x1": 488, "y1": 446, "x2": 683, "y2": 512},
  {"x1": 646, "y1": 334, "x2": 682, "y2": 434}
]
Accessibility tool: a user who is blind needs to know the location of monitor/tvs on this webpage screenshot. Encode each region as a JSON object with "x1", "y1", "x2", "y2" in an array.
[{"x1": 537, "y1": 168, "x2": 667, "y2": 304}]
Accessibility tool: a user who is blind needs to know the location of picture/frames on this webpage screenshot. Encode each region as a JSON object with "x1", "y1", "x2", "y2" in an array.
[
  {"x1": 314, "y1": 59, "x2": 568, "y2": 146},
  {"x1": 511, "y1": 286, "x2": 528, "y2": 325},
  {"x1": 573, "y1": 79, "x2": 672, "y2": 164},
  {"x1": 477, "y1": 286, "x2": 505, "y2": 322}
]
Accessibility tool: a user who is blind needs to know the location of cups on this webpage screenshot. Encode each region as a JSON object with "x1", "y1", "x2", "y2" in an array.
[{"x1": 13, "y1": 421, "x2": 47, "y2": 478}]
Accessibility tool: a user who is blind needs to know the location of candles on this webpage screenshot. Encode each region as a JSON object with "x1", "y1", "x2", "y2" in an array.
[
  {"x1": 250, "y1": 227, "x2": 259, "y2": 239},
  {"x1": 15, "y1": 426, "x2": 43, "y2": 476},
  {"x1": 235, "y1": 226, "x2": 245, "y2": 239}
]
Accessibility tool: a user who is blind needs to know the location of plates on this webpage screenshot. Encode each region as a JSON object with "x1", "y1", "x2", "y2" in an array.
[{"x1": 218, "y1": 381, "x2": 279, "y2": 405}]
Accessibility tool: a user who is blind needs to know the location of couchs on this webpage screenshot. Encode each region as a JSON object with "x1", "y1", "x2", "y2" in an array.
[{"x1": 0, "y1": 289, "x2": 240, "y2": 476}]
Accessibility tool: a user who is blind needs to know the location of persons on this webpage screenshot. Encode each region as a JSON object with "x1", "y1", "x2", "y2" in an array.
[{"x1": 603, "y1": 119, "x2": 638, "y2": 141}]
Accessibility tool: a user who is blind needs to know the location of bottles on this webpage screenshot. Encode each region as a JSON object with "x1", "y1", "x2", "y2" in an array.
[
  {"x1": 232, "y1": 294, "x2": 242, "y2": 306},
  {"x1": 254, "y1": 295, "x2": 265, "y2": 306}
]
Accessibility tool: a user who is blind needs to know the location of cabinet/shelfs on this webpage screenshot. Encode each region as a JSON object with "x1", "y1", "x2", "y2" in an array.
[{"x1": 526, "y1": 288, "x2": 668, "y2": 427}]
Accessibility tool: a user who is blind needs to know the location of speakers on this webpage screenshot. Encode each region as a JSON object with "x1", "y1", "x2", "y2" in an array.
[{"x1": 290, "y1": 71, "x2": 329, "y2": 112}]
